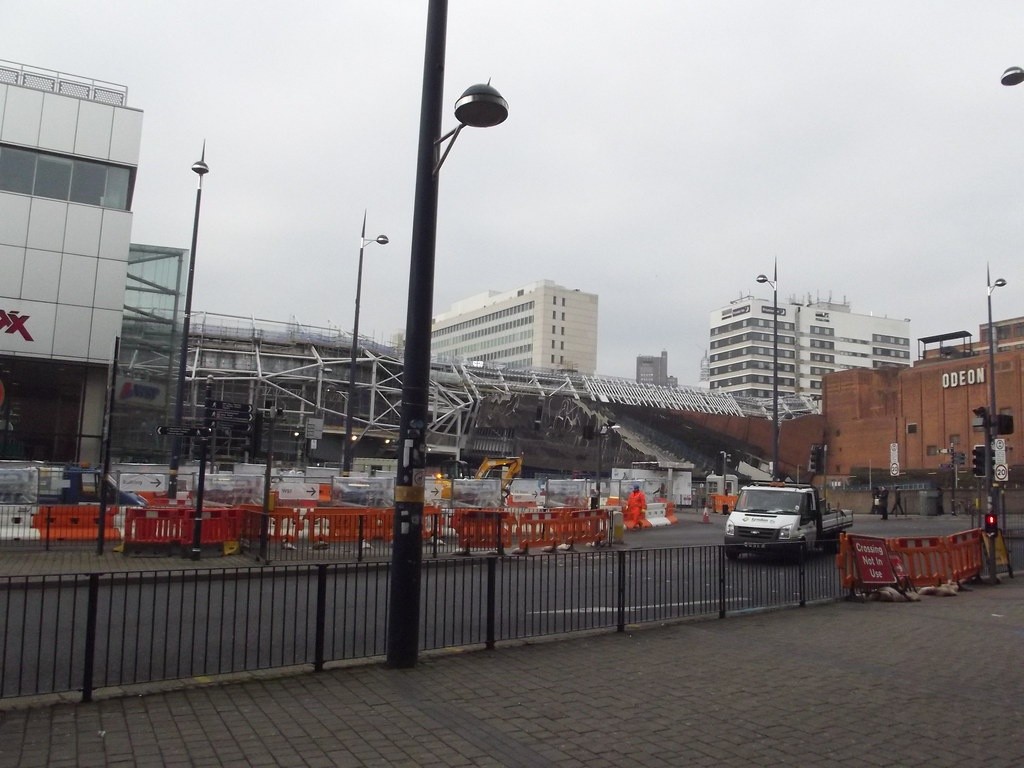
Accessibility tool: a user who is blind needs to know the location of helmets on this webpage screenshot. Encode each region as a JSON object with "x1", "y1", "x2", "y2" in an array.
[{"x1": 634, "y1": 484, "x2": 639, "y2": 490}]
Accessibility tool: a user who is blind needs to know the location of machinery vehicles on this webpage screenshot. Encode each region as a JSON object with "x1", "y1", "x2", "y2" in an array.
[{"x1": 434, "y1": 456, "x2": 522, "y2": 506}]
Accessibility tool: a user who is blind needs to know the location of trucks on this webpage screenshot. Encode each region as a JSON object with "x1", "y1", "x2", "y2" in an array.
[{"x1": 0, "y1": 462, "x2": 149, "y2": 507}]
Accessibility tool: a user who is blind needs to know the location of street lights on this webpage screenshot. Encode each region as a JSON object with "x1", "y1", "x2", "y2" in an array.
[
  {"x1": 1001, "y1": 66, "x2": 1024, "y2": 86},
  {"x1": 987, "y1": 261, "x2": 1007, "y2": 434},
  {"x1": 374, "y1": 0, "x2": 509, "y2": 669},
  {"x1": 343, "y1": 209, "x2": 389, "y2": 477},
  {"x1": 167, "y1": 138, "x2": 209, "y2": 500},
  {"x1": 756, "y1": 258, "x2": 778, "y2": 476}
]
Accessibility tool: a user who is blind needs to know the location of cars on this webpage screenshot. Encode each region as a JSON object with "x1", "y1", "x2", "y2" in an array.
[{"x1": 333, "y1": 477, "x2": 376, "y2": 501}]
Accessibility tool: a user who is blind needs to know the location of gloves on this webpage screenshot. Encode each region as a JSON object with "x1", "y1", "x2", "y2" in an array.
[
  {"x1": 642, "y1": 509, "x2": 645, "y2": 513},
  {"x1": 626, "y1": 506, "x2": 628, "y2": 510}
]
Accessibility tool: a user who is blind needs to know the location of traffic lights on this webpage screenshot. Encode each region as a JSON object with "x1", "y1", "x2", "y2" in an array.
[
  {"x1": 972, "y1": 444, "x2": 986, "y2": 477},
  {"x1": 583, "y1": 426, "x2": 594, "y2": 439},
  {"x1": 985, "y1": 514, "x2": 998, "y2": 538}
]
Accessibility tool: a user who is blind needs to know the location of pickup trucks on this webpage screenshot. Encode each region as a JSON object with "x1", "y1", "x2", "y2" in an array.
[{"x1": 724, "y1": 481, "x2": 853, "y2": 559}]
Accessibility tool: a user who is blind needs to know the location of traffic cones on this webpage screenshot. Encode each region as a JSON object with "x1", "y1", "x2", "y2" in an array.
[{"x1": 701, "y1": 507, "x2": 712, "y2": 524}]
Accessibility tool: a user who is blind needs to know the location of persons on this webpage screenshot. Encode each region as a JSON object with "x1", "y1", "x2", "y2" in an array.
[
  {"x1": 869, "y1": 485, "x2": 905, "y2": 520},
  {"x1": 624, "y1": 484, "x2": 646, "y2": 531},
  {"x1": 654, "y1": 483, "x2": 668, "y2": 499},
  {"x1": 540, "y1": 489, "x2": 547, "y2": 496}
]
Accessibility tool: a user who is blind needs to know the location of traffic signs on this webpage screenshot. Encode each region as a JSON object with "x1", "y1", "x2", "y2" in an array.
[
  {"x1": 939, "y1": 448, "x2": 967, "y2": 468},
  {"x1": 156, "y1": 398, "x2": 254, "y2": 438}
]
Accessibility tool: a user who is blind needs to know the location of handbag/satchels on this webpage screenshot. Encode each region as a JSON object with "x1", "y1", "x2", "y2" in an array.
[{"x1": 875, "y1": 499, "x2": 879, "y2": 505}]
